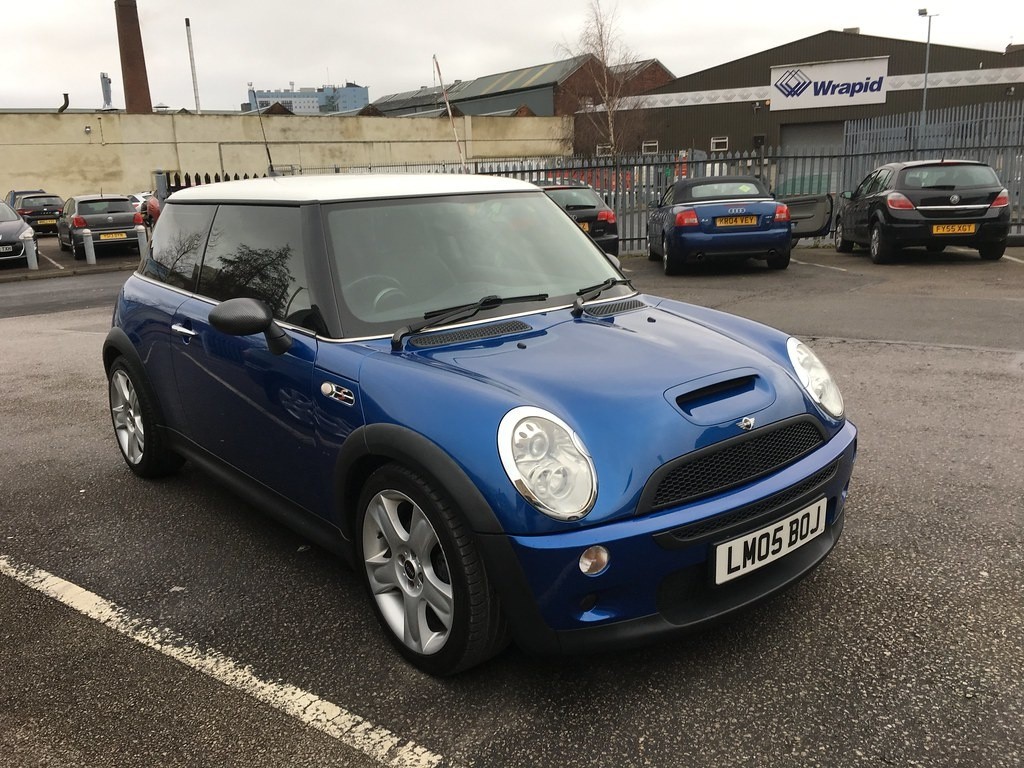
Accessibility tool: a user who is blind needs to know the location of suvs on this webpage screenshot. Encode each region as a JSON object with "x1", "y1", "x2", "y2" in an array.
[
  {"x1": 125, "y1": 190, "x2": 159, "y2": 213},
  {"x1": 5, "y1": 189, "x2": 46, "y2": 207}
]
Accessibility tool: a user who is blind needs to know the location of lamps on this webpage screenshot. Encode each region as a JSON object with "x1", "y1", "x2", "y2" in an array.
[{"x1": 1006, "y1": 86, "x2": 1015, "y2": 96}]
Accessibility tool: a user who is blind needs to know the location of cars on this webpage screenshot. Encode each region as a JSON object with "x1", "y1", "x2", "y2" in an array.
[
  {"x1": 103, "y1": 175, "x2": 858, "y2": 682},
  {"x1": 835, "y1": 159, "x2": 1011, "y2": 266},
  {"x1": 153, "y1": 183, "x2": 196, "y2": 202},
  {"x1": 474, "y1": 186, "x2": 619, "y2": 262},
  {"x1": 524, "y1": 177, "x2": 593, "y2": 190},
  {"x1": 12, "y1": 194, "x2": 65, "y2": 231},
  {"x1": 0, "y1": 199, "x2": 40, "y2": 268},
  {"x1": 643, "y1": 177, "x2": 832, "y2": 276},
  {"x1": 52, "y1": 194, "x2": 147, "y2": 261}
]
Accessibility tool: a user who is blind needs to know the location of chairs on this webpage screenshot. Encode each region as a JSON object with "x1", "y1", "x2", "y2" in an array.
[{"x1": 368, "y1": 206, "x2": 455, "y2": 309}]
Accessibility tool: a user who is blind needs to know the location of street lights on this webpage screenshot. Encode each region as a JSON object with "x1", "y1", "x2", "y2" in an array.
[{"x1": 917, "y1": 8, "x2": 932, "y2": 110}]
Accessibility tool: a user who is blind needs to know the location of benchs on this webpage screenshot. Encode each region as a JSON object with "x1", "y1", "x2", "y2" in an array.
[{"x1": 908, "y1": 176, "x2": 977, "y2": 187}]
